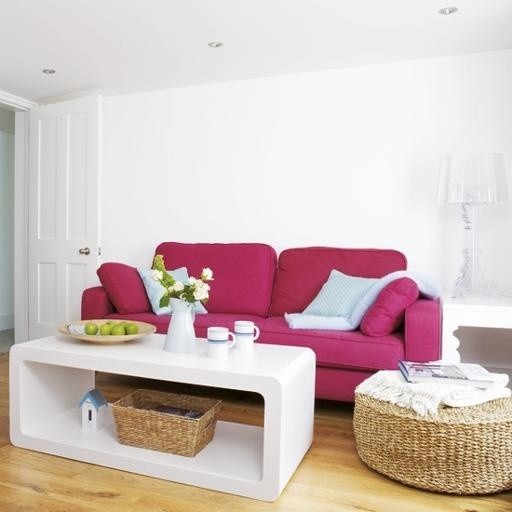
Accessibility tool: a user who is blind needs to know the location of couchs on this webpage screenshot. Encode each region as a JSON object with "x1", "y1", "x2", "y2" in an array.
[{"x1": 82, "y1": 284, "x2": 442, "y2": 405}]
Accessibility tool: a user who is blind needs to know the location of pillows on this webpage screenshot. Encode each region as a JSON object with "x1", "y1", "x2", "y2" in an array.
[
  {"x1": 138, "y1": 266, "x2": 208, "y2": 317},
  {"x1": 270, "y1": 248, "x2": 407, "y2": 318},
  {"x1": 360, "y1": 279, "x2": 418, "y2": 338},
  {"x1": 97, "y1": 262, "x2": 151, "y2": 314},
  {"x1": 153, "y1": 242, "x2": 276, "y2": 321}
]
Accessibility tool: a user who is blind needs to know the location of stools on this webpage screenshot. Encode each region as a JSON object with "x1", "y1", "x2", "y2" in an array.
[{"x1": 352, "y1": 372, "x2": 512, "y2": 495}]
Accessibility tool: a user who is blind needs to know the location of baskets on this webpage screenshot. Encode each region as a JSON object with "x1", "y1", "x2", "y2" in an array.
[{"x1": 111, "y1": 387, "x2": 223, "y2": 457}]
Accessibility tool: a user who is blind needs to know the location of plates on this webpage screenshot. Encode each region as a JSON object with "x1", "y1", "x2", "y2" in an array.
[{"x1": 59, "y1": 319, "x2": 157, "y2": 345}]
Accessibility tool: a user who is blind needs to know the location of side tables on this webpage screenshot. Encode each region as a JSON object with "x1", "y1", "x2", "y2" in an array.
[{"x1": 440, "y1": 295, "x2": 512, "y2": 370}]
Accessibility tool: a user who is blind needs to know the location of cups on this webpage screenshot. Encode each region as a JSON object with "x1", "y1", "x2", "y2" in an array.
[
  {"x1": 234, "y1": 320, "x2": 260, "y2": 349},
  {"x1": 207, "y1": 326, "x2": 236, "y2": 358}
]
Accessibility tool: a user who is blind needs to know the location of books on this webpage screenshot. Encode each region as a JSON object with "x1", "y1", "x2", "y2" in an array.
[{"x1": 397, "y1": 359, "x2": 495, "y2": 383}]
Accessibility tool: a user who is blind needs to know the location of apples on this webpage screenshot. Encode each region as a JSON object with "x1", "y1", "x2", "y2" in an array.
[{"x1": 85, "y1": 322, "x2": 138, "y2": 335}]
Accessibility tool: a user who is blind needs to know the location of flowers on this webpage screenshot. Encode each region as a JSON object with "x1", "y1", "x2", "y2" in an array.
[{"x1": 152, "y1": 254, "x2": 214, "y2": 309}]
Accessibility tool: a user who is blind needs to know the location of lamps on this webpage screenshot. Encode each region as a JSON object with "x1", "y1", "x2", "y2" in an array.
[{"x1": 436, "y1": 152, "x2": 508, "y2": 299}]
